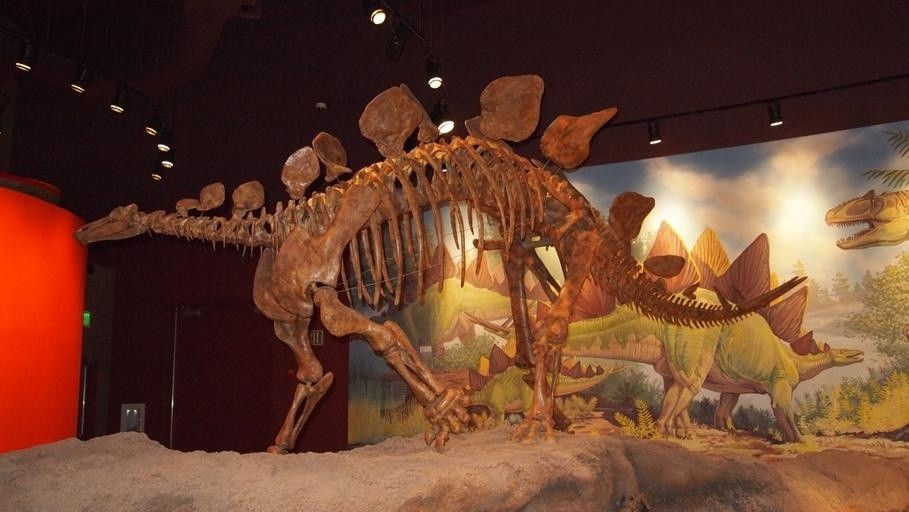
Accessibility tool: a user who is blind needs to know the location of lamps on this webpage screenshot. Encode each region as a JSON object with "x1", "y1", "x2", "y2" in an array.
[
  {"x1": 645, "y1": 96, "x2": 786, "y2": 146},
  {"x1": 362, "y1": 1, "x2": 458, "y2": 134},
  {"x1": 13, "y1": 37, "x2": 179, "y2": 181}
]
